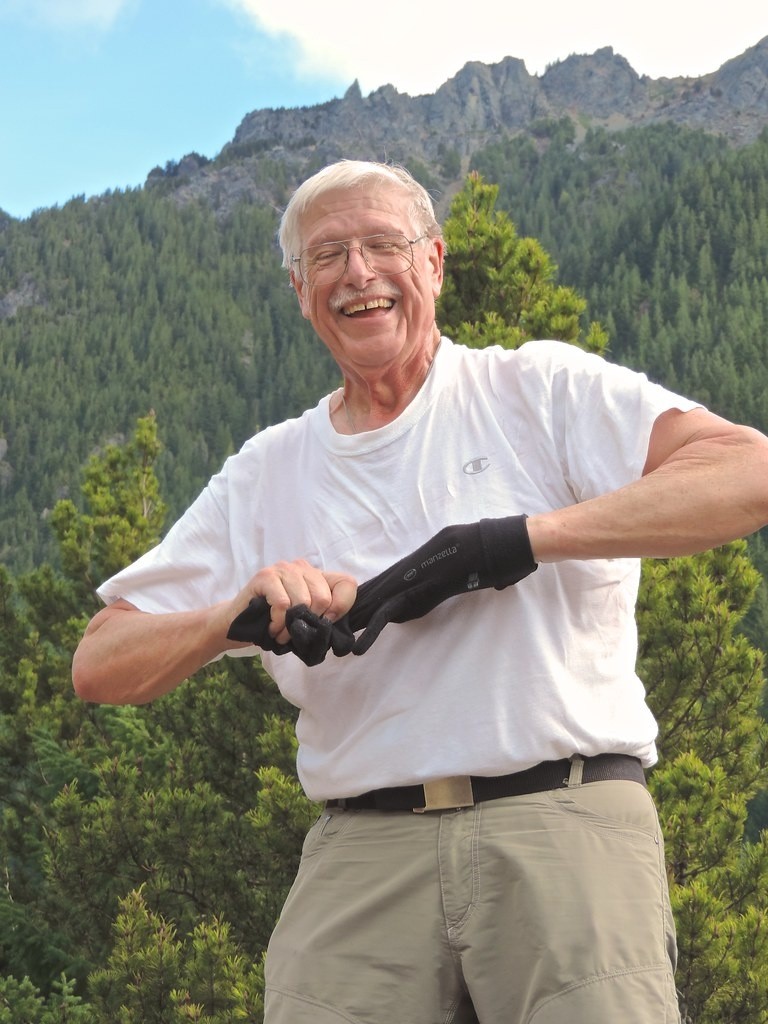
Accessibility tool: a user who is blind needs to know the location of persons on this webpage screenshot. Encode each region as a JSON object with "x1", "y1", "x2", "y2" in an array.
[{"x1": 70, "y1": 158, "x2": 767, "y2": 1023}]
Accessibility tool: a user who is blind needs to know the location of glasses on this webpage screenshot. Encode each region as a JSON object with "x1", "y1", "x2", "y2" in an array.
[{"x1": 291, "y1": 234, "x2": 427, "y2": 285}]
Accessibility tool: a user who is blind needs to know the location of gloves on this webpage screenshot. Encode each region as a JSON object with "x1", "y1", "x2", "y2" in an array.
[
  {"x1": 226, "y1": 596, "x2": 355, "y2": 667},
  {"x1": 348, "y1": 514, "x2": 538, "y2": 656}
]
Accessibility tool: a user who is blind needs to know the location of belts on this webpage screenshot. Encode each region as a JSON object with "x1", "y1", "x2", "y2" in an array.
[{"x1": 326, "y1": 753, "x2": 645, "y2": 813}]
